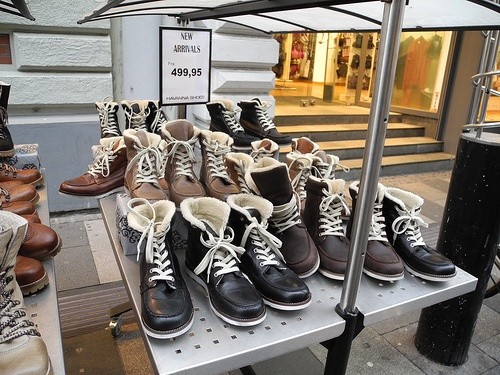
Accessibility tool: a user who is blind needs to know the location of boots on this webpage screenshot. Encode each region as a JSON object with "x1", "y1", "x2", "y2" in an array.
[
  {"x1": 303, "y1": 175, "x2": 351, "y2": 280},
  {"x1": 346, "y1": 181, "x2": 405, "y2": 281},
  {"x1": 382, "y1": 187, "x2": 458, "y2": 282},
  {"x1": 179, "y1": 197, "x2": 268, "y2": 327},
  {"x1": 206, "y1": 99, "x2": 261, "y2": 150},
  {"x1": 0, "y1": 82, "x2": 62, "y2": 375},
  {"x1": 147, "y1": 100, "x2": 169, "y2": 132},
  {"x1": 127, "y1": 197, "x2": 194, "y2": 339},
  {"x1": 160, "y1": 118, "x2": 206, "y2": 212},
  {"x1": 95, "y1": 95, "x2": 122, "y2": 137},
  {"x1": 58, "y1": 136, "x2": 128, "y2": 199},
  {"x1": 226, "y1": 194, "x2": 312, "y2": 311},
  {"x1": 236, "y1": 98, "x2": 293, "y2": 147},
  {"x1": 313, "y1": 151, "x2": 354, "y2": 221},
  {"x1": 123, "y1": 128, "x2": 170, "y2": 207},
  {"x1": 287, "y1": 151, "x2": 324, "y2": 216},
  {"x1": 120, "y1": 99, "x2": 150, "y2": 129},
  {"x1": 244, "y1": 157, "x2": 321, "y2": 279},
  {"x1": 198, "y1": 130, "x2": 241, "y2": 200}
]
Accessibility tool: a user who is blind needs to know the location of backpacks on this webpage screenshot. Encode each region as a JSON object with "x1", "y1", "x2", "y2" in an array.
[{"x1": 334, "y1": 32, "x2": 375, "y2": 90}]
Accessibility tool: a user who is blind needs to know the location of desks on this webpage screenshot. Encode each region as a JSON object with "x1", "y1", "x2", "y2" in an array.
[
  {"x1": 0, "y1": 168, "x2": 65, "y2": 375},
  {"x1": 98, "y1": 189, "x2": 477, "y2": 375}
]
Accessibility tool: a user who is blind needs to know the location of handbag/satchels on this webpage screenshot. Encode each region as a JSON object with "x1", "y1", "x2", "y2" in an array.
[{"x1": 272, "y1": 32, "x2": 310, "y2": 80}]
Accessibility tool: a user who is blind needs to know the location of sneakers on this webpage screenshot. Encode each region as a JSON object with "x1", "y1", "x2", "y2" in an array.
[
  {"x1": 224, "y1": 152, "x2": 255, "y2": 195},
  {"x1": 248, "y1": 136, "x2": 279, "y2": 161}
]
